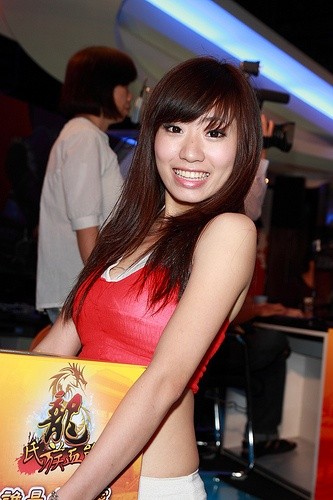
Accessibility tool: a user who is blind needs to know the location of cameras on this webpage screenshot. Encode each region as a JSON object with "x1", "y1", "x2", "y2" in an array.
[{"x1": 262, "y1": 121, "x2": 296, "y2": 151}]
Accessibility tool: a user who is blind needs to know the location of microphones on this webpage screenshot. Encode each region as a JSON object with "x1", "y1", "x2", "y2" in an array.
[{"x1": 254, "y1": 89, "x2": 289, "y2": 104}]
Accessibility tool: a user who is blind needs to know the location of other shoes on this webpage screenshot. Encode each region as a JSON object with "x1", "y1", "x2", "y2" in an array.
[{"x1": 241, "y1": 438, "x2": 296, "y2": 457}]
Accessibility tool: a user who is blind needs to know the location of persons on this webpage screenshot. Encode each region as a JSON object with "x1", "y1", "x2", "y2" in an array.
[
  {"x1": 31, "y1": 57, "x2": 263, "y2": 500},
  {"x1": 37, "y1": 45, "x2": 138, "y2": 327},
  {"x1": 244, "y1": 114, "x2": 273, "y2": 221},
  {"x1": 4, "y1": 118, "x2": 56, "y2": 271},
  {"x1": 209, "y1": 219, "x2": 297, "y2": 458},
  {"x1": 288, "y1": 238, "x2": 321, "y2": 312}
]
